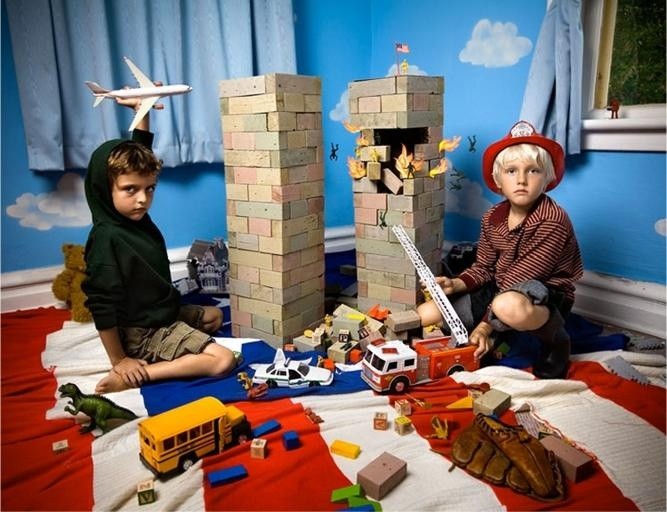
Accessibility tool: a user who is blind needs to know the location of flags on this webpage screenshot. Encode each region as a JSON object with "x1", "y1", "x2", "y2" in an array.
[{"x1": 396, "y1": 43, "x2": 411, "y2": 53}]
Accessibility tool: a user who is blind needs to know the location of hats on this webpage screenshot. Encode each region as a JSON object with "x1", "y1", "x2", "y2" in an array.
[{"x1": 483, "y1": 121, "x2": 565, "y2": 195}]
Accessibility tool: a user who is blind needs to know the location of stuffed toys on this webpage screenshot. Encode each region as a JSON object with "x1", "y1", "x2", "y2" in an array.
[{"x1": 49, "y1": 242, "x2": 91, "y2": 327}]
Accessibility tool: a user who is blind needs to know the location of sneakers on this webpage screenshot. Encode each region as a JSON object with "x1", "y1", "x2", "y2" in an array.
[{"x1": 533, "y1": 351, "x2": 568, "y2": 379}]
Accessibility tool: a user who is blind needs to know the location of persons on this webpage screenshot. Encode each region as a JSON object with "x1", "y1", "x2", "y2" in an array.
[
  {"x1": 416, "y1": 118, "x2": 585, "y2": 380},
  {"x1": 80, "y1": 79, "x2": 239, "y2": 396}
]
